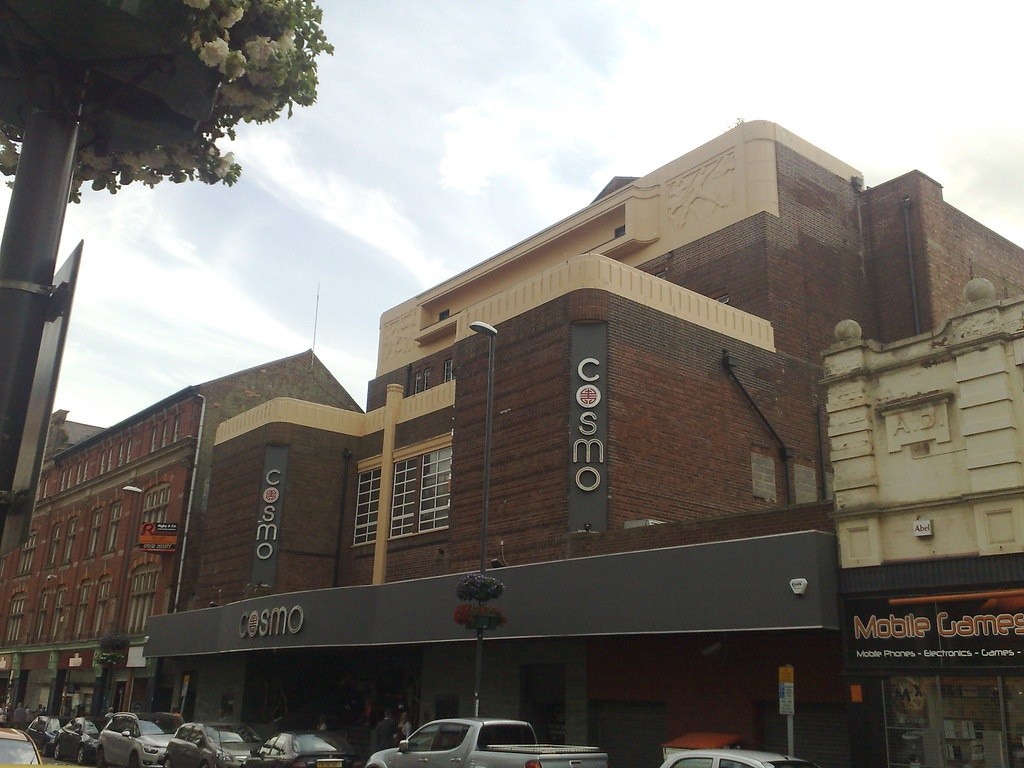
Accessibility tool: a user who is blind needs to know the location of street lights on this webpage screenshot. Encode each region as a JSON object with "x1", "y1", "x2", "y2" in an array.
[
  {"x1": 471, "y1": 320, "x2": 499, "y2": 717},
  {"x1": 107, "y1": 484, "x2": 142, "y2": 710}
]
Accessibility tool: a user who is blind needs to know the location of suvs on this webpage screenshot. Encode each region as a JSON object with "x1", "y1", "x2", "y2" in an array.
[{"x1": 98, "y1": 711, "x2": 185, "y2": 768}]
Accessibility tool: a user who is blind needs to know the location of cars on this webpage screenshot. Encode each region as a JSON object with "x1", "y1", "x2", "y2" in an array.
[
  {"x1": 660, "y1": 750, "x2": 820, "y2": 768},
  {"x1": 240, "y1": 728, "x2": 363, "y2": 768},
  {"x1": 55, "y1": 716, "x2": 112, "y2": 765},
  {"x1": 27, "y1": 715, "x2": 75, "y2": 756},
  {"x1": 163, "y1": 720, "x2": 264, "y2": 768},
  {"x1": 0, "y1": 728, "x2": 43, "y2": 766}
]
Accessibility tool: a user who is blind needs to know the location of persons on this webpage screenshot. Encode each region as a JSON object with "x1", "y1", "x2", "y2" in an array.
[
  {"x1": 315, "y1": 717, "x2": 326, "y2": 730},
  {"x1": 105, "y1": 706, "x2": 115, "y2": 719},
  {"x1": 171, "y1": 708, "x2": 182, "y2": 717},
  {"x1": 0, "y1": 700, "x2": 70, "y2": 731},
  {"x1": 396, "y1": 712, "x2": 412, "y2": 746}
]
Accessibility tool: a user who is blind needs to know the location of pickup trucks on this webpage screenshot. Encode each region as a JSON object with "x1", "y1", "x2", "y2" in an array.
[{"x1": 366, "y1": 717, "x2": 608, "y2": 768}]
